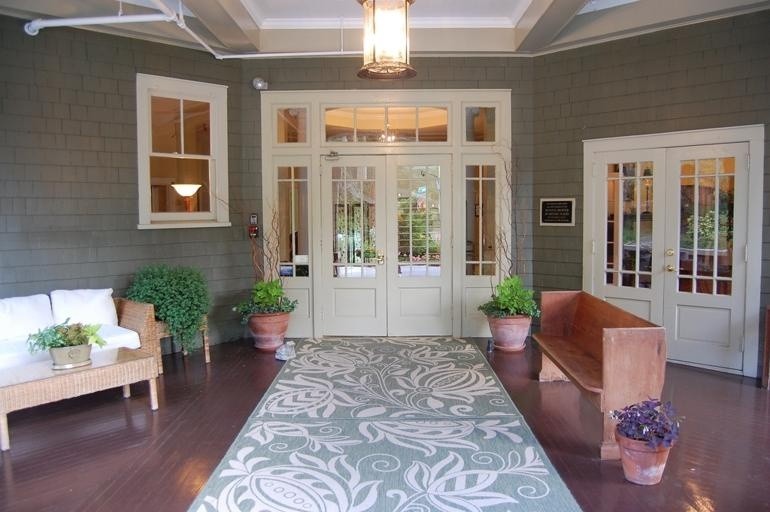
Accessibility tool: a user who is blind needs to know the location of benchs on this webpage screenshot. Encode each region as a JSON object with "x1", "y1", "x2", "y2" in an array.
[
  {"x1": 530, "y1": 289, "x2": 669, "y2": 462},
  {"x1": 0, "y1": 297, "x2": 161, "y2": 452}
]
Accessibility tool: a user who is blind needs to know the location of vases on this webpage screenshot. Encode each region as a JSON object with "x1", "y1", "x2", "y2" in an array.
[{"x1": 614, "y1": 428, "x2": 674, "y2": 486}]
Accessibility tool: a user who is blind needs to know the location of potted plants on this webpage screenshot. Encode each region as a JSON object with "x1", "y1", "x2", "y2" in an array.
[
  {"x1": 124, "y1": 264, "x2": 212, "y2": 365},
  {"x1": 183, "y1": 335, "x2": 585, "y2": 512},
  {"x1": 233, "y1": 278, "x2": 299, "y2": 350},
  {"x1": 476, "y1": 273, "x2": 540, "y2": 353},
  {"x1": 24, "y1": 317, "x2": 107, "y2": 371}
]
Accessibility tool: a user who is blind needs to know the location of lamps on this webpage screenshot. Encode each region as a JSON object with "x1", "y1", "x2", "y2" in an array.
[
  {"x1": 355, "y1": 0, "x2": 418, "y2": 81},
  {"x1": 170, "y1": 182, "x2": 203, "y2": 213}
]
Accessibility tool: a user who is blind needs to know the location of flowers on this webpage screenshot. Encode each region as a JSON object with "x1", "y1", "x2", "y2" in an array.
[{"x1": 609, "y1": 397, "x2": 690, "y2": 449}]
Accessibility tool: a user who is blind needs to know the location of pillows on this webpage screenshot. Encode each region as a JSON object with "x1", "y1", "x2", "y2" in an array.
[{"x1": 1, "y1": 287, "x2": 142, "y2": 366}]
